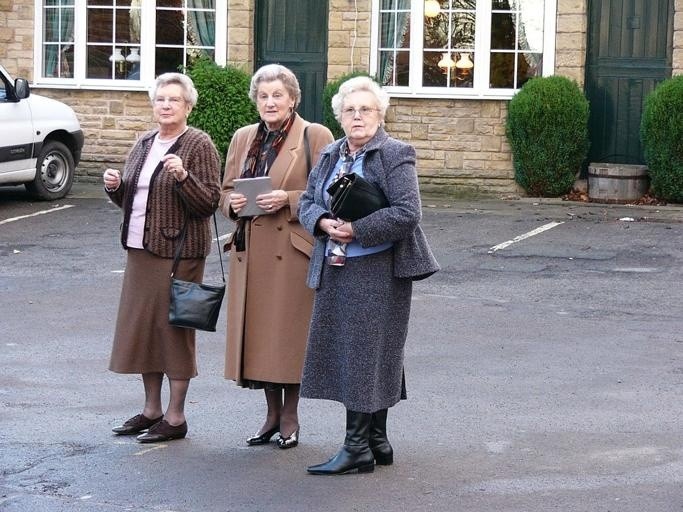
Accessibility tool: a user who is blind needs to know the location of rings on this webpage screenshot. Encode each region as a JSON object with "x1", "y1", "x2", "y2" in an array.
[
  {"x1": 174, "y1": 169, "x2": 177, "y2": 172},
  {"x1": 268, "y1": 204, "x2": 272, "y2": 210}
]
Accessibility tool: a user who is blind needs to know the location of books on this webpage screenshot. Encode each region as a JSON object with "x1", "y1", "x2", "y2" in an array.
[{"x1": 232, "y1": 176, "x2": 274, "y2": 218}]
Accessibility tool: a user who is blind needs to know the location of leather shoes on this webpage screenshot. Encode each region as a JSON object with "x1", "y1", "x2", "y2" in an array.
[
  {"x1": 137, "y1": 420, "x2": 187, "y2": 443},
  {"x1": 112, "y1": 414, "x2": 164, "y2": 434}
]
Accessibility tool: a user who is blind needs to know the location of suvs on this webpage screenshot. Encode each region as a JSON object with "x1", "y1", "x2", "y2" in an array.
[{"x1": 0, "y1": 65, "x2": 84, "y2": 200}]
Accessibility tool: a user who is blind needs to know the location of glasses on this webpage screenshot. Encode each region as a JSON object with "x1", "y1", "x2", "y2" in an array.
[{"x1": 341, "y1": 107, "x2": 382, "y2": 115}]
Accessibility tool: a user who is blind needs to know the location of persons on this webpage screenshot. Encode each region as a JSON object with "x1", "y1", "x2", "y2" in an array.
[
  {"x1": 220, "y1": 64, "x2": 336, "y2": 449},
  {"x1": 100, "y1": 70, "x2": 223, "y2": 445},
  {"x1": 295, "y1": 75, "x2": 439, "y2": 476}
]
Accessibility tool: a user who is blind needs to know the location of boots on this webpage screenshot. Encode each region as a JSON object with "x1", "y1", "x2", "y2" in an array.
[
  {"x1": 369, "y1": 408, "x2": 393, "y2": 465},
  {"x1": 308, "y1": 408, "x2": 374, "y2": 476}
]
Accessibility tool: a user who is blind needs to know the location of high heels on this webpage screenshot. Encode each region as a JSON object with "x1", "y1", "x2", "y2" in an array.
[
  {"x1": 246, "y1": 421, "x2": 280, "y2": 445},
  {"x1": 276, "y1": 424, "x2": 299, "y2": 449}
]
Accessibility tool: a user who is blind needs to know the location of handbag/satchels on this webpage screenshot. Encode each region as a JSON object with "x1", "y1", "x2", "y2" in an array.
[
  {"x1": 326, "y1": 172, "x2": 389, "y2": 222},
  {"x1": 169, "y1": 280, "x2": 225, "y2": 333}
]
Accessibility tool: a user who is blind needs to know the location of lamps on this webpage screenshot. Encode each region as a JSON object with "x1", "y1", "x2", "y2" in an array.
[
  {"x1": 438, "y1": 53, "x2": 474, "y2": 87},
  {"x1": 108, "y1": 48, "x2": 140, "y2": 80}
]
